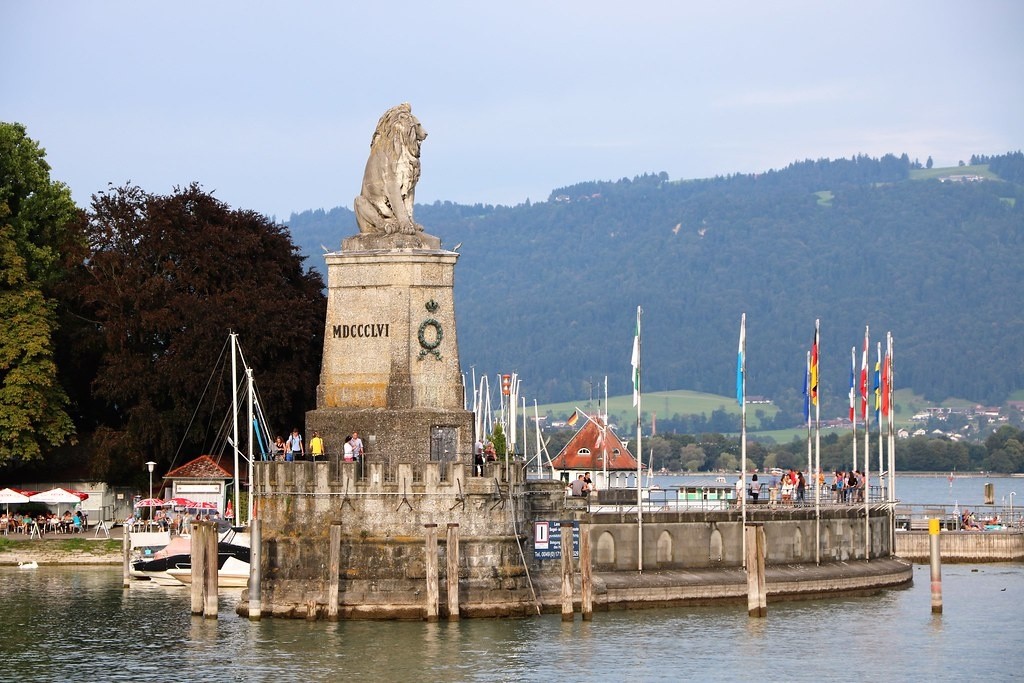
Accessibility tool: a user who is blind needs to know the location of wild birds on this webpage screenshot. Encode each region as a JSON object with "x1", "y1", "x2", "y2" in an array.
[{"x1": 18, "y1": 559, "x2": 38, "y2": 569}]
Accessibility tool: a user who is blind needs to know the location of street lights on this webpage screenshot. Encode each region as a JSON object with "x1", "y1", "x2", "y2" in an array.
[{"x1": 145, "y1": 461, "x2": 157, "y2": 533}]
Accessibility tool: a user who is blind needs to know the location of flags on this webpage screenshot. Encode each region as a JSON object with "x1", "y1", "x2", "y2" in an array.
[
  {"x1": 810, "y1": 329, "x2": 817, "y2": 406},
  {"x1": 850, "y1": 355, "x2": 853, "y2": 421},
  {"x1": 631, "y1": 324, "x2": 638, "y2": 408},
  {"x1": 874, "y1": 354, "x2": 879, "y2": 421},
  {"x1": 882, "y1": 357, "x2": 889, "y2": 416},
  {"x1": 736, "y1": 329, "x2": 743, "y2": 407},
  {"x1": 859, "y1": 339, "x2": 866, "y2": 419},
  {"x1": 804, "y1": 367, "x2": 808, "y2": 422}
]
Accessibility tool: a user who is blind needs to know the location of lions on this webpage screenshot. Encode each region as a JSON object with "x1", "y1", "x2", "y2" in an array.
[{"x1": 354, "y1": 102, "x2": 429, "y2": 236}]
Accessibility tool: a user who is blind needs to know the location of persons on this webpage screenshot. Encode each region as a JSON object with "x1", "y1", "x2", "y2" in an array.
[
  {"x1": 275, "y1": 428, "x2": 305, "y2": 461},
  {"x1": 475, "y1": 439, "x2": 484, "y2": 477},
  {"x1": 568, "y1": 472, "x2": 592, "y2": 497},
  {"x1": 484, "y1": 442, "x2": 497, "y2": 461},
  {"x1": 344, "y1": 436, "x2": 357, "y2": 461},
  {"x1": 960, "y1": 509, "x2": 1001, "y2": 531},
  {"x1": 750, "y1": 474, "x2": 760, "y2": 503},
  {"x1": 768, "y1": 468, "x2": 805, "y2": 505},
  {"x1": 309, "y1": 431, "x2": 327, "y2": 461},
  {"x1": 831, "y1": 471, "x2": 865, "y2": 503},
  {"x1": 814, "y1": 467, "x2": 825, "y2": 503},
  {"x1": 349, "y1": 432, "x2": 366, "y2": 469},
  {"x1": 736, "y1": 475, "x2": 743, "y2": 509},
  {"x1": 125, "y1": 509, "x2": 222, "y2": 534},
  {"x1": 0, "y1": 512, "x2": 85, "y2": 534}
]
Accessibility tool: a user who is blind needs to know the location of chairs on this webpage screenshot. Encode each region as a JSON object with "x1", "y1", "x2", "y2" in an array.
[{"x1": 0, "y1": 518, "x2": 84, "y2": 536}]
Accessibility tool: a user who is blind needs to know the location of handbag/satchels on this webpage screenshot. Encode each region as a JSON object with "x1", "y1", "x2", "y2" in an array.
[
  {"x1": 748, "y1": 489, "x2": 751, "y2": 495},
  {"x1": 486, "y1": 455, "x2": 494, "y2": 461},
  {"x1": 791, "y1": 493, "x2": 797, "y2": 501},
  {"x1": 832, "y1": 484, "x2": 836, "y2": 491}
]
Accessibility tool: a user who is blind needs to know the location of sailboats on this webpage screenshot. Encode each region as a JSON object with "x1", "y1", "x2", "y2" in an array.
[
  {"x1": 130, "y1": 332, "x2": 271, "y2": 587},
  {"x1": 984, "y1": 483, "x2": 994, "y2": 504}
]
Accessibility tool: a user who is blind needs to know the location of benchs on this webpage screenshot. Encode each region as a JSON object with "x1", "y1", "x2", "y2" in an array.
[{"x1": 910, "y1": 518, "x2": 929, "y2": 532}]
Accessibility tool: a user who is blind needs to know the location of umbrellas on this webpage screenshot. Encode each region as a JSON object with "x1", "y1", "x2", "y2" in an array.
[
  {"x1": 0, "y1": 488, "x2": 89, "y2": 522},
  {"x1": 134, "y1": 498, "x2": 235, "y2": 522}
]
